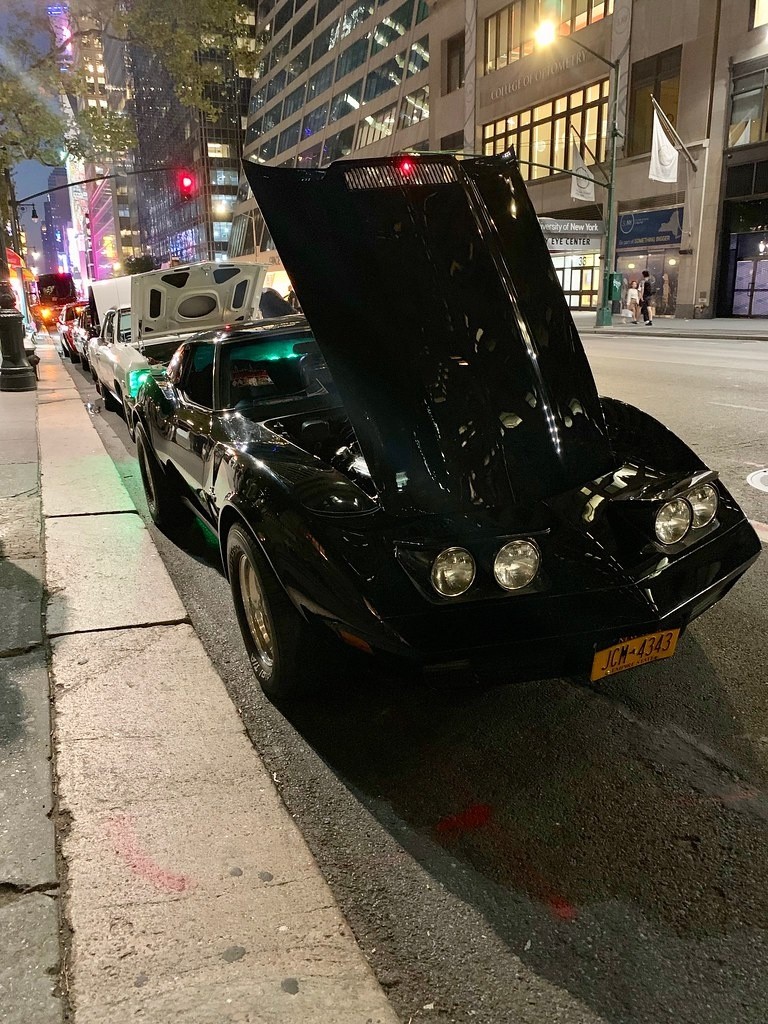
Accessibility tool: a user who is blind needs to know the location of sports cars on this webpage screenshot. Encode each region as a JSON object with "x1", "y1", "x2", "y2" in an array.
[{"x1": 126, "y1": 144, "x2": 760, "y2": 720}]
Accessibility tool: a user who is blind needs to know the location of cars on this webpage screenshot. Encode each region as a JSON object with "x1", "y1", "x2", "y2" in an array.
[
  {"x1": 71, "y1": 273, "x2": 138, "y2": 382},
  {"x1": 85, "y1": 258, "x2": 270, "y2": 444},
  {"x1": 40, "y1": 299, "x2": 100, "y2": 371}
]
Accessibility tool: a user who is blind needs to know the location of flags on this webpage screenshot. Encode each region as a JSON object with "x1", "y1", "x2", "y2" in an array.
[
  {"x1": 570, "y1": 136, "x2": 595, "y2": 203},
  {"x1": 648, "y1": 109, "x2": 679, "y2": 183}
]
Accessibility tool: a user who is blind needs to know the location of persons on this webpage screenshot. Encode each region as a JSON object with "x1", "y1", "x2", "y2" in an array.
[{"x1": 621, "y1": 270, "x2": 652, "y2": 326}]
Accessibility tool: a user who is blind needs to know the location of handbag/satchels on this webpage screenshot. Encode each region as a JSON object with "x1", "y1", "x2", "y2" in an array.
[{"x1": 621, "y1": 305, "x2": 633, "y2": 318}]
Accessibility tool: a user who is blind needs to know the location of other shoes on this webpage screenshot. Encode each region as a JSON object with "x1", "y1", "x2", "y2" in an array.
[
  {"x1": 645, "y1": 321, "x2": 652, "y2": 325},
  {"x1": 633, "y1": 321, "x2": 638, "y2": 324}
]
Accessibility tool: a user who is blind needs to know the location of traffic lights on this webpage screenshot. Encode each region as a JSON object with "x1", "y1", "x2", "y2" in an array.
[{"x1": 179, "y1": 172, "x2": 194, "y2": 202}]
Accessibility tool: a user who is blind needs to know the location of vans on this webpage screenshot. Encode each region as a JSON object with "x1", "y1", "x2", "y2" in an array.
[{"x1": 35, "y1": 268, "x2": 82, "y2": 330}]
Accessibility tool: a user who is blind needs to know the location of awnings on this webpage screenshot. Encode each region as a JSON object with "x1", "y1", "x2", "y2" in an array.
[
  {"x1": 538, "y1": 217, "x2": 605, "y2": 251},
  {"x1": 6, "y1": 248, "x2": 36, "y2": 282}
]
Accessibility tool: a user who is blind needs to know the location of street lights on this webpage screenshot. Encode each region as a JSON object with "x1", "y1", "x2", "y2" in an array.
[
  {"x1": 214, "y1": 204, "x2": 257, "y2": 253},
  {"x1": 14, "y1": 202, "x2": 40, "y2": 311},
  {"x1": 534, "y1": 19, "x2": 621, "y2": 328}
]
